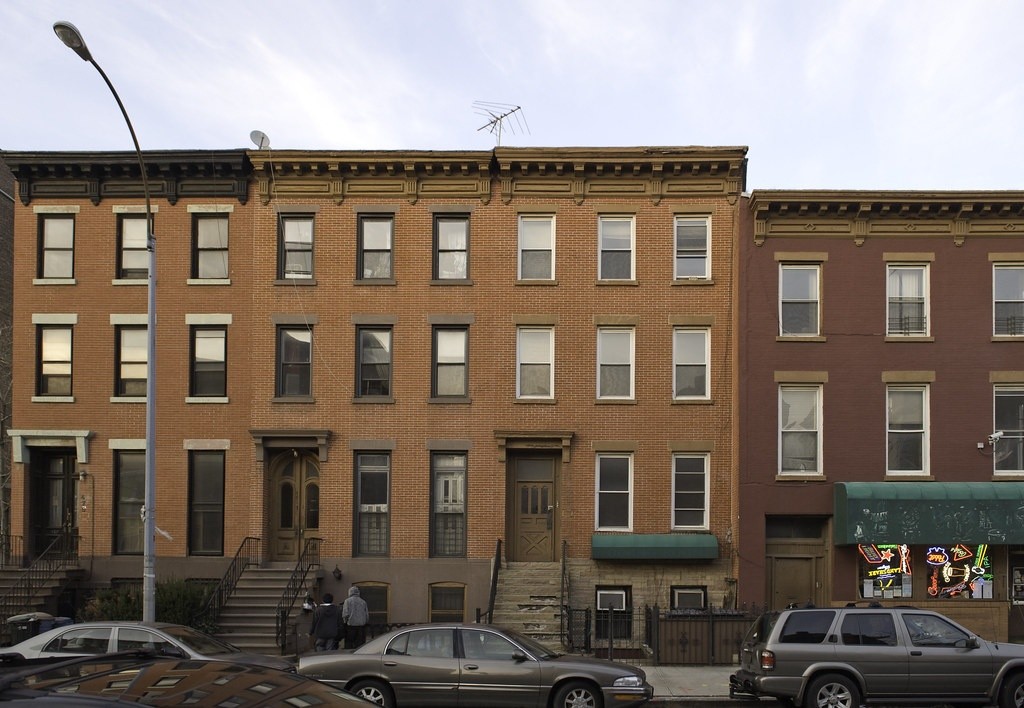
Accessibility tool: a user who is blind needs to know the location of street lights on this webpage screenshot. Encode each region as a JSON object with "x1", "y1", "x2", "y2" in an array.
[{"x1": 52, "y1": 20, "x2": 157, "y2": 625}]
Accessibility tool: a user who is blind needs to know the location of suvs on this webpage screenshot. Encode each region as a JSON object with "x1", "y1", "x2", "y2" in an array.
[{"x1": 728, "y1": 598, "x2": 1023, "y2": 708}]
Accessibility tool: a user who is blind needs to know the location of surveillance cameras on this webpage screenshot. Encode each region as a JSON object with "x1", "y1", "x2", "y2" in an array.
[{"x1": 989, "y1": 432, "x2": 1004, "y2": 440}]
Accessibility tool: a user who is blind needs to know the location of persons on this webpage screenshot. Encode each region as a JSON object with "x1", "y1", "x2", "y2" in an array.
[{"x1": 308, "y1": 586, "x2": 369, "y2": 652}]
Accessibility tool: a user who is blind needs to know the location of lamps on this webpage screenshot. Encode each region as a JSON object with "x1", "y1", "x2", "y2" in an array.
[{"x1": 332, "y1": 564, "x2": 342, "y2": 579}]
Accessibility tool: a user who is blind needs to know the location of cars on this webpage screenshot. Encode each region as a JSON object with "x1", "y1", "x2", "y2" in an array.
[
  {"x1": 0, "y1": 621, "x2": 297, "y2": 680},
  {"x1": 0, "y1": 651, "x2": 386, "y2": 708},
  {"x1": 296, "y1": 623, "x2": 654, "y2": 708}
]
